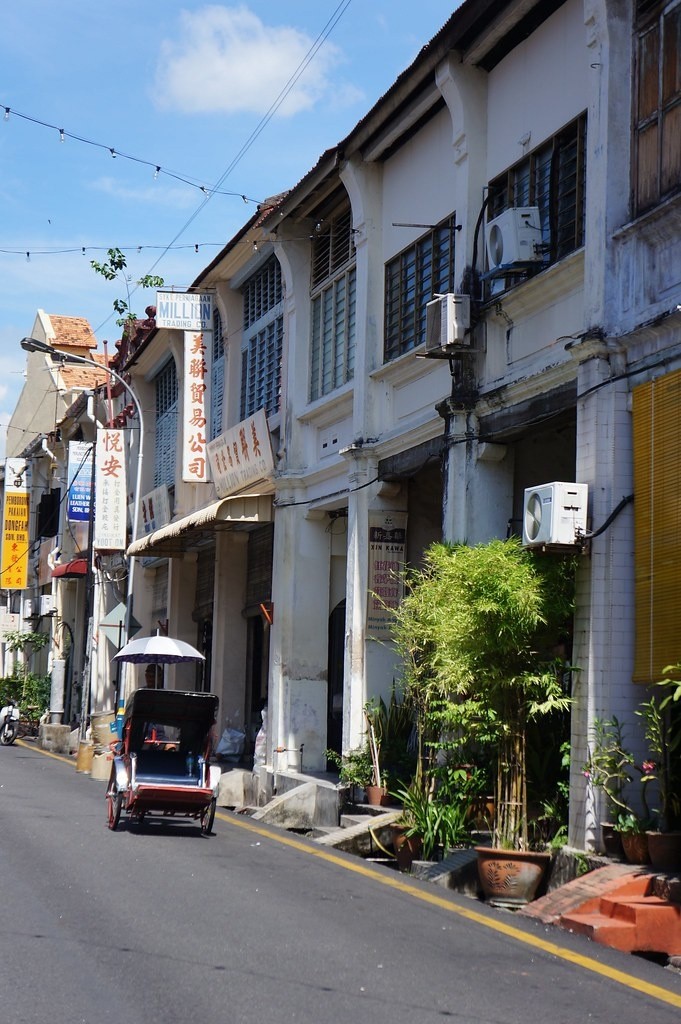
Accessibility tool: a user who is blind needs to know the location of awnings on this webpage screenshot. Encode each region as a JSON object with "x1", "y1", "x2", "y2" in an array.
[
  {"x1": 51, "y1": 558, "x2": 88, "y2": 578},
  {"x1": 126, "y1": 492, "x2": 275, "y2": 559}
]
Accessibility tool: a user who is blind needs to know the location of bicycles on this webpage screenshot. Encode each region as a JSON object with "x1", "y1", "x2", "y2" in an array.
[{"x1": 16, "y1": 705, "x2": 51, "y2": 739}]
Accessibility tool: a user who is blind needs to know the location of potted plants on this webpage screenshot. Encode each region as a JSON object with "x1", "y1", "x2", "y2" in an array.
[
  {"x1": 648, "y1": 663, "x2": 681, "y2": 873},
  {"x1": 321, "y1": 534, "x2": 576, "y2": 909}
]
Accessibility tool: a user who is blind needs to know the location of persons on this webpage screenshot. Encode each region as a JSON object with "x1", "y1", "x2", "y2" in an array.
[{"x1": 137, "y1": 664, "x2": 162, "y2": 689}]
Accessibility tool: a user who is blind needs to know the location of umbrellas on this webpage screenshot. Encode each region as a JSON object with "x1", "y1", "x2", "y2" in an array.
[{"x1": 110, "y1": 628, "x2": 206, "y2": 689}]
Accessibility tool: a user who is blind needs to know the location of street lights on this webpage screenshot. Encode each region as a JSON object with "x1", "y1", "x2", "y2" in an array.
[{"x1": 20, "y1": 337, "x2": 145, "y2": 746}]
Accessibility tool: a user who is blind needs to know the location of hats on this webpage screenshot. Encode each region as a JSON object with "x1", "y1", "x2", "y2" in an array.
[{"x1": 145, "y1": 664, "x2": 163, "y2": 674}]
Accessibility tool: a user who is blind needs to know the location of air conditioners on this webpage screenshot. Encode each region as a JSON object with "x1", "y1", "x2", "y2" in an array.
[
  {"x1": 522, "y1": 481, "x2": 587, "y2": 551},
  {"x1": 485, "y1": 205, "x2": 541, "y2": 270},
  {"x1": 425, "y1": 292, "x2": 471, "y2": 354}
]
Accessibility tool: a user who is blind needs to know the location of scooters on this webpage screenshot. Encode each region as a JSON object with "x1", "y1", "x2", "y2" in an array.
[{"x1": 0, "y1": 687, "x2": 27, "y2": 746}]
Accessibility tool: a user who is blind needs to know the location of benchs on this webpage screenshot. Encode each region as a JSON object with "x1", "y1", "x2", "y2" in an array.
[{"x1": 133, "y1": 747, "x2": 201, "y2": 785}]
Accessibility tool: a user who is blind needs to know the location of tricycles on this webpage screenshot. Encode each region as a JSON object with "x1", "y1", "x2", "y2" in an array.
[{"x1": 104, "y1": 689, "x2": 222, "y2": 835}]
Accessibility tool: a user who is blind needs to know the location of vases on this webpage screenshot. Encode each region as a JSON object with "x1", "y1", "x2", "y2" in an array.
[
  {"x1": 599, "y1": 820, "x2": 621, "y2": 856},
  {"x1": 620, "y1": 823, "x2": 648, "y2": 865}
]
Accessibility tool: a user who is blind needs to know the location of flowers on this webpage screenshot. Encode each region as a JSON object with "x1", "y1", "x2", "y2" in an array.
[{"x1": 579, "y1": 710, "x2": 662, "y2": 830}]
